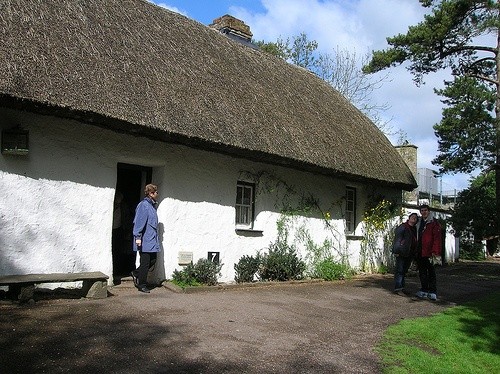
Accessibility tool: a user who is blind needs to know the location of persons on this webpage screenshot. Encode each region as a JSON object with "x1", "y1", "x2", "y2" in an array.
[
  {"x1": 416, "y1": 204, "x2": 441, "y2": 301},
  {"x1": 130, "y1": 183, "x2": 160, "y2": 293},
  {"x1": 391, "y1": 212, "x2": 419, "y2": 297}
]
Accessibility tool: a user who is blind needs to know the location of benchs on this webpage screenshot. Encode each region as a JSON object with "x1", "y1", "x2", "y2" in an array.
[{"x1": 0, "y1": 271, "x2": 109, "y2": 305}]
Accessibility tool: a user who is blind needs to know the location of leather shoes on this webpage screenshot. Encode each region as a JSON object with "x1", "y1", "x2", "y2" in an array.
[
  {"x1": 138, "y1": 285, "x2": 150, "y2": 293},
  {"x1": 130, "y1": 271, "x2": 137, "y2": 286}
]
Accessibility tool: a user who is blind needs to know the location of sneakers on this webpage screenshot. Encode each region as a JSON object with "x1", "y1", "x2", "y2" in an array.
[
  {"x1": 416, "y1": 291, "x2": 427, "y2": 297},
  {"x1": 430, "y1": 293, "x2": 436, "y2": 299}
]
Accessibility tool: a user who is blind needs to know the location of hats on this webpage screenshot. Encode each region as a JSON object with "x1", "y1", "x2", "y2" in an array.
[{"x1": 144, "y1": 184, "x2": 157, "y2": 192}]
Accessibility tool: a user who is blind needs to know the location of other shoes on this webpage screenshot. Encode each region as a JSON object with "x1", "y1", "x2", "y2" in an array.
[{"x1": 395, "y1": 290, "x2": 408, "y2": 295}]
